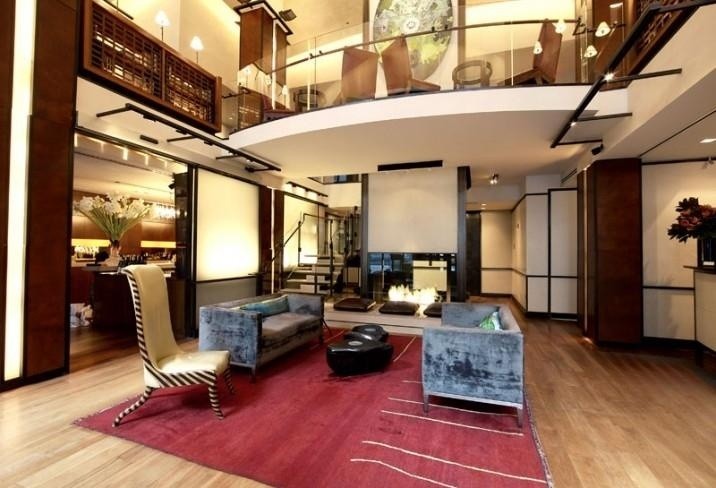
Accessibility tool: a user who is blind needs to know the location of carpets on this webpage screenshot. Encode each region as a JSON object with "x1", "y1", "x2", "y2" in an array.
[{"x1": 71, "y1": 324, "x2": 556, "y2": 487}]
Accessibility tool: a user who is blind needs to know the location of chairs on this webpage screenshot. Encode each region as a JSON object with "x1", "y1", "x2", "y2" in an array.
[
  {"x1": 496, "y1": 18, "x2": 563, "y2": 86},
  {"x1": 111, "y1": 263, "x2": 235, "y2": 427},
  {"x1": 331, "y1": 32, "x2": 441, "y2": 109},
  {"x1": 411, "y1": 260, "x2": 452, "y2": 305}
]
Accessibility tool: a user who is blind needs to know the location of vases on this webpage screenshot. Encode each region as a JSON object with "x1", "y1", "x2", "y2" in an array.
[{"x1": 104, "y1": 242, "x2": 123, "y2": 267}]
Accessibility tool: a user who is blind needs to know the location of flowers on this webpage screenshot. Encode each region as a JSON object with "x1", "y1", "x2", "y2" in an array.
[
  {"x1": 666, "y1": 194, "x2": 715, "y2": 244},
  {"x1": 72, "y1": 192, "x2": 157, "y2": 245}
]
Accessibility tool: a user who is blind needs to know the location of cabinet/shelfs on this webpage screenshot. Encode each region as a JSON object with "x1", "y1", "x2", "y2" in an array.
[{"x1": 77, "y1": 0, "x2": 222, "y2": 135}]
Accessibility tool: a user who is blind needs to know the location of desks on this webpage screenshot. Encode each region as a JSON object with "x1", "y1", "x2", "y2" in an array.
[
  {"x1": 453, "y1": 58, "x2": 494, "y2": 90},
  {"x1": 292, "y1": 88, "x2": 327, "y2": 113}
]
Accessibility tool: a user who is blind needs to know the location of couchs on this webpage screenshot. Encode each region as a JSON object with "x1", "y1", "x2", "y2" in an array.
[
  {"x1": 198, "y1": 290, "x2": 328, "y2": 384},
  {"x1": 420, "y1": 303, "x2": 525, "y2": 427}
]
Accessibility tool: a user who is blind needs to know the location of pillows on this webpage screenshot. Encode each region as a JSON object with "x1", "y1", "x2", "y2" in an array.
[{"x1": 472, "y1": 311, "x2": 505, "y2": 332}]
